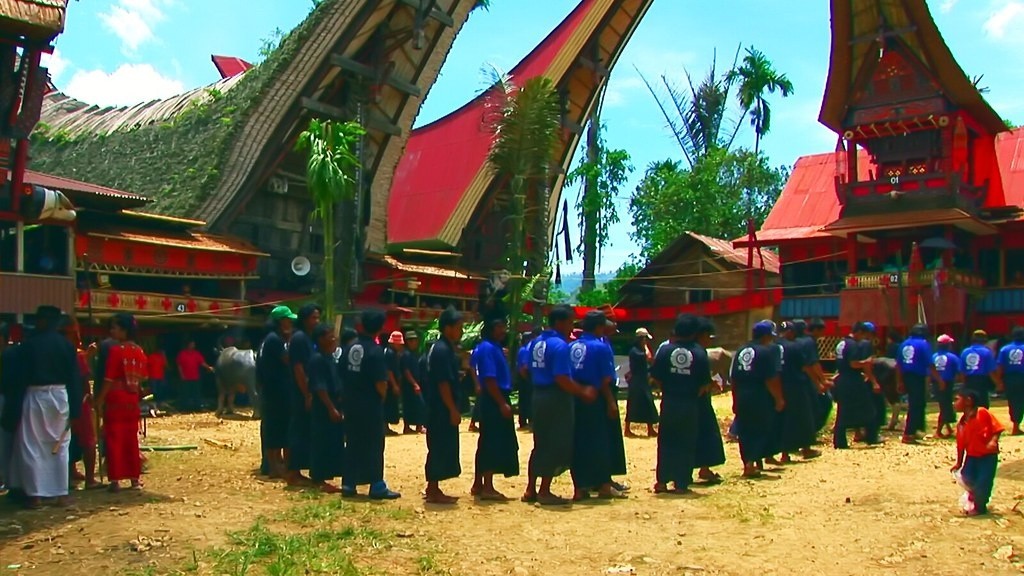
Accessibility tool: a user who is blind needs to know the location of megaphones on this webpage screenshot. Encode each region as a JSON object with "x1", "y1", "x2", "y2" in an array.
[{"x1": 291, "y1": 256, "x2": 310, "y2": 275}]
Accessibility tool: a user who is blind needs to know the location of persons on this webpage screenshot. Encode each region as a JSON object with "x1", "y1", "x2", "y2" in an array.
[
  {"x1": 949, "y1": 390, "x2": 1006, "y2": 517},
  {"x1": 421, "y1": 309, "x2": 466, "y2": 506},
  {"x1": 0, "y1": 300, "x2": 1023, "y2": 505},
  {"x1": 624, "y1": 327, "x2": 660, "y2": 438}
]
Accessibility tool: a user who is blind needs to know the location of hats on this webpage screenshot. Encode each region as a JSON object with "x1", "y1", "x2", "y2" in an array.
[
  {"x1": 973, "y1": 330, "x2": 987, "y2": 341},
  {"x1": 388, "y1": 331, "x2": 404, "y2": 344},
  {"x1": 938, "y1": 335, "x2": 955, "y2": 344},
  {"x1": 271, "y1": 306, "x2": 298, "y2": 319},
  {"x1": 752, "y1": 322, "x2": 777, "y2": 337},
  {"x1": 779, "y1": 321, "x2": 793, "y2": 331},
  {"x1": 636, "y1": 327, "x2": 653, "y2": 340},
  {"x1": 586, "y1": 310, "x2": 614, "y2": 328},
  {"x1": 760, "y1": 320, "x2": 778, "y2": 336},
  {"x1": 863, "y1": 322, "x2": 875, "y2": 332}
]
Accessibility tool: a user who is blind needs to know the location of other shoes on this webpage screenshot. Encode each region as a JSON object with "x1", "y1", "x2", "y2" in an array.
[
  {"x1": 0, "y1": 474, "x2": 142, "y2": 509},
  {"x1": 834, "y1": 419, "x2": 954, "y2": 448},
  {"x1": 260, "y1": 422, "x2": 823, "y2": 505},
  {"x1": 964, "y1": 492, "x2": 979, "y2": 515}
]
